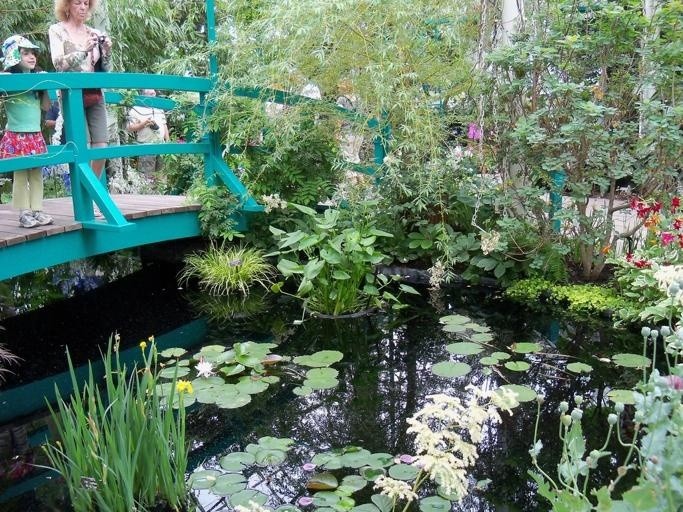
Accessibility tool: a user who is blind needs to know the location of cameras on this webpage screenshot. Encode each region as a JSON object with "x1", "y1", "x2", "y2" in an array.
[
  {"x1": 95, "y1": 34, "x2": 107, "y2": 43},
  {"x1": 150, "y1": 122, "x2": 160, "y2": 130}
]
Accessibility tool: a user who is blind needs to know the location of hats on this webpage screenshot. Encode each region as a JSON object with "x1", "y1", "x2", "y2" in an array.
[{"x1": 0, "y1": 35, "x2": 40, "y2": 71}]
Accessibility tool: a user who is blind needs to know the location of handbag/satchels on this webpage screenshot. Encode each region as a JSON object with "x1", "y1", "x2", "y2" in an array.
[{"x1": 83, "y1": 89, "x2": 102, "y2": 108}]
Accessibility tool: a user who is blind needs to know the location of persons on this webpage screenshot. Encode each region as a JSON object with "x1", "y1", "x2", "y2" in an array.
[
  {"x1": 336, "y1": 76, "x2": 368, "y2": 189},
  {"x1": 44, "y1": 89, "x2": 73, "y2": 192},
  {"x1": 2, "y1": 34, "x2": 54, "y2": 229},
  {"x1": 48, "y1": 0, "x2": 114, "y2": 218},
  {"x1": 125, "y1": 89, "x2": 172, "y2": 183}
]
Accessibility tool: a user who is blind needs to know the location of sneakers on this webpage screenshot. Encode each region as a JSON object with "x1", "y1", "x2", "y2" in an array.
[
  {"x1": 94, "y1": 208, "x2": 101, "y2": 217},
  {"x1": 18, "y1": 211, "x2": 53, "y2": 228}
]
